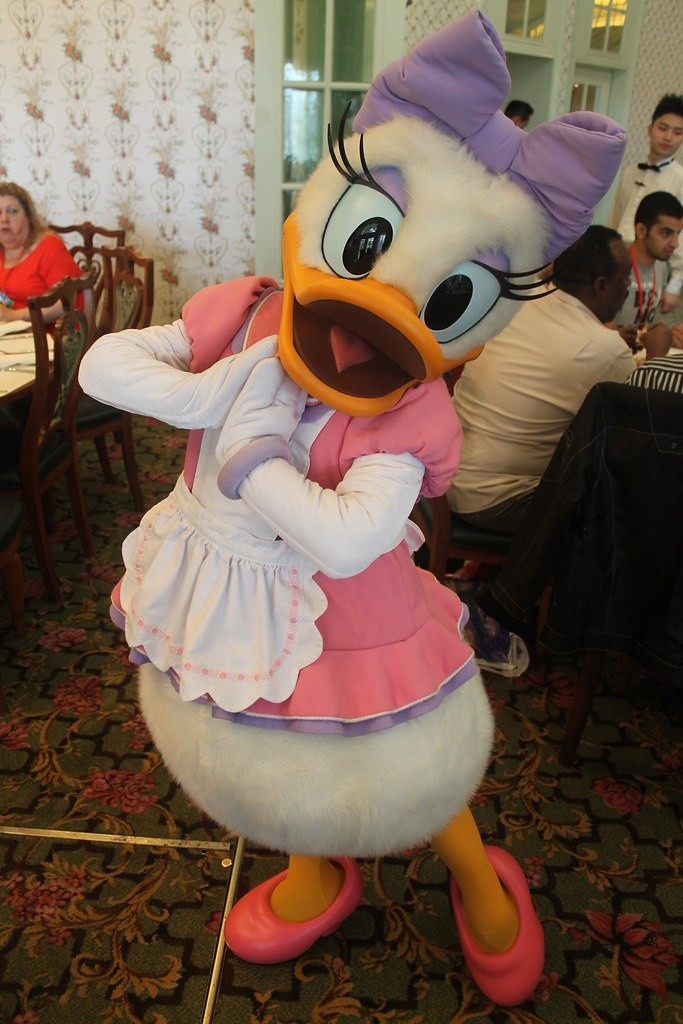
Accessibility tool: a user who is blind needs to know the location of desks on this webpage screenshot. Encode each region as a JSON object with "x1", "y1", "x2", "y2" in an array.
[{"x1": 0, "y1": 317, "x2": 57, "y2": 402}]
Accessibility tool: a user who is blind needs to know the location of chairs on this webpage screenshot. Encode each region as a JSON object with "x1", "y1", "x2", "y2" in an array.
[
  {"x1": 409, "y1": 493, "x2": 683, "y2": 765},
  {"x1": 0, "y1": 221, "x2": 154, "y2": 640}
]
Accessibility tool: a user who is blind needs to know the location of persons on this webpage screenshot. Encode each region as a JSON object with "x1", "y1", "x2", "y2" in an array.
[
  {"x1": 504, "y1": 99, "x2": 535, "y2": 130},
  {"x1": 613, "y1": 191, "x2": 683, "y2": 327},
  {"x1": 0, "y1": 183, "x2": 84, "y2": 338},
  {"x1": 605, "y1": 91, "x2": 683, "y2": 314},
  {"x1": 446, "y1": 225, "x2": 672, "y2": 538}
]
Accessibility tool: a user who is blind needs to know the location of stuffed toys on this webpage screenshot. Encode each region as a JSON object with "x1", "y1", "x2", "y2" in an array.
[{"x1": 77, "y1": 7, "x2": 629, "y2": 1009}]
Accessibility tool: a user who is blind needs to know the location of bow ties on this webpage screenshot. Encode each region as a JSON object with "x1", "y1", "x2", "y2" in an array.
[{"x1": 636, "y1": 161, "x2": 662, "y2": 173}]
